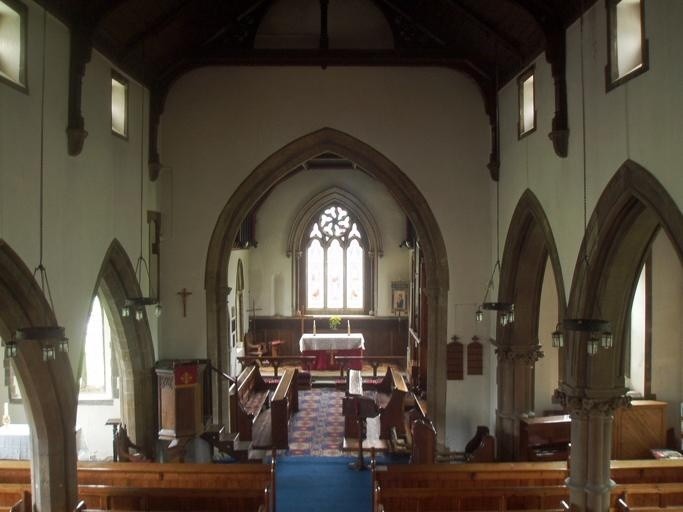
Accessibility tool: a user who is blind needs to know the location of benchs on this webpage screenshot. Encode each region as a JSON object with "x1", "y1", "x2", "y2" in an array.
[
  {"x1": 374, "y1": 364, "x2": 408, "y2": 440},
  {"x1": 230, "y1": 364, "x2": 271, "y2": 441}
]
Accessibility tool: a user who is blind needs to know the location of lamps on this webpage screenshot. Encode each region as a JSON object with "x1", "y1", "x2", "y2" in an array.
[
  {"x1": 476, "y1": 42, "x2": 515, "y2": 329},
  {"x1": 122, "y1": 64, "x2": 163, "y2": 321},
  {"x1": 552, "y1": 12, "x2": 613, "y2": 355},
  {"x1": 6, "y1": 6, "x2": 70, "y2": 361}
]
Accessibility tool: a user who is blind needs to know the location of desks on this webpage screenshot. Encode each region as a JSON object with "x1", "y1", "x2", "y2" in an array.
[
  {"x1": 299, "y1": 334, "x2": 366, "y2": 370},
  {"x1": 0, "y1": 423, "x2": 81, "y2": 459}
]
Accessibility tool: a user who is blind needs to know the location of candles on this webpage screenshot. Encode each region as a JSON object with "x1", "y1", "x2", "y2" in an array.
[
  {"x1": 348, "y1": 320, "x2": 350, "y2": 327},
  {"x1": 4, "y1": 402, "x2": 8, "y2": 418},
  {"x1": 313, "y1": 320, "x2": 316, "y2": 327}
]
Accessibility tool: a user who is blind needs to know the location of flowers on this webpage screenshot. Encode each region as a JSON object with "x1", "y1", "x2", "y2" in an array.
[{"x1": 328, "y1": 316, "x2": 342, "y2": 329}]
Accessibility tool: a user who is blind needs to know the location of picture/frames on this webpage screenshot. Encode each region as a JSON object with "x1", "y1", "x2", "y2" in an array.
[{"x1": 391, "y1": 287, "x2": 408, "y2": 314}]
